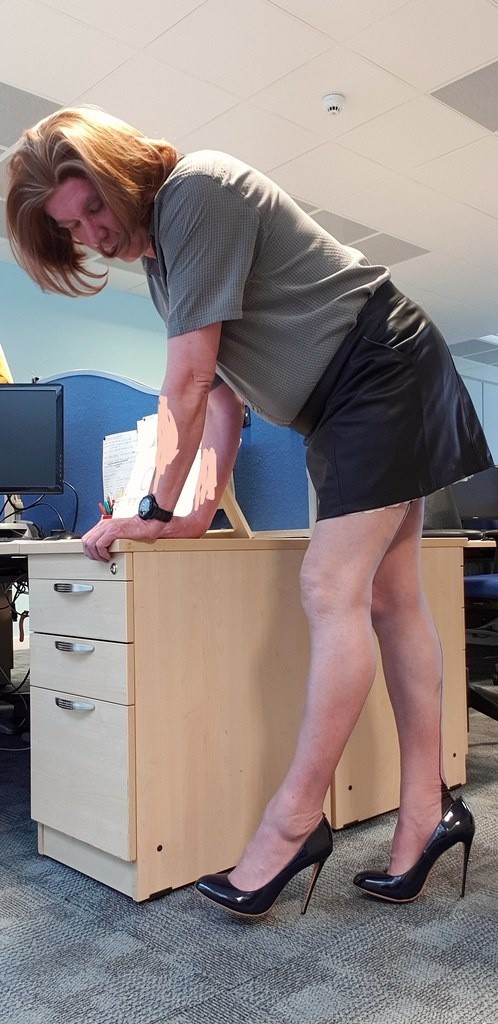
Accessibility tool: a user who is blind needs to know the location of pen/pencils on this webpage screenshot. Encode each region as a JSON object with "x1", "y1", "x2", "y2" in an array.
[{"x1": 97, "y1": 496, "x2": 115, "y2": 519}]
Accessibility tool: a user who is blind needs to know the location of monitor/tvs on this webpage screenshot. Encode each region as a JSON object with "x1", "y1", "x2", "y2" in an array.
[
  {"x1": 452, "y1": 465, "x2": 498, "y2": 520},
  {"x1": 0, "y1": 383, "x2": 64, "y2": 495}
]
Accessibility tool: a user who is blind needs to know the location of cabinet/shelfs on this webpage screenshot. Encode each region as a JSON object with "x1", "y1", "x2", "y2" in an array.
[{"x1": 26, "y1": 541, "x2": 336, "y2": 904}]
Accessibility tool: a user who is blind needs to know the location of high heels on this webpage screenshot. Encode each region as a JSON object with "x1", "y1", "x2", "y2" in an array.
[
  {"x1": 193, "y1": 810, "x2": 333, "y2": 916},
  {"x1": 353, "y1": 796, "x2": 476, "y2": 903}
]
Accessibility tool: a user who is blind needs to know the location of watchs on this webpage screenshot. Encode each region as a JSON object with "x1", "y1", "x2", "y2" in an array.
[{"x1": 137, "y1": 493, "x2": 174, "y2": 522}]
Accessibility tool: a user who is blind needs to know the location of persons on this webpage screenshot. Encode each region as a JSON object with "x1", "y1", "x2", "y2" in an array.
[{"x1": 3, "y1": 104, "x2": 496, "y2": 924}]
